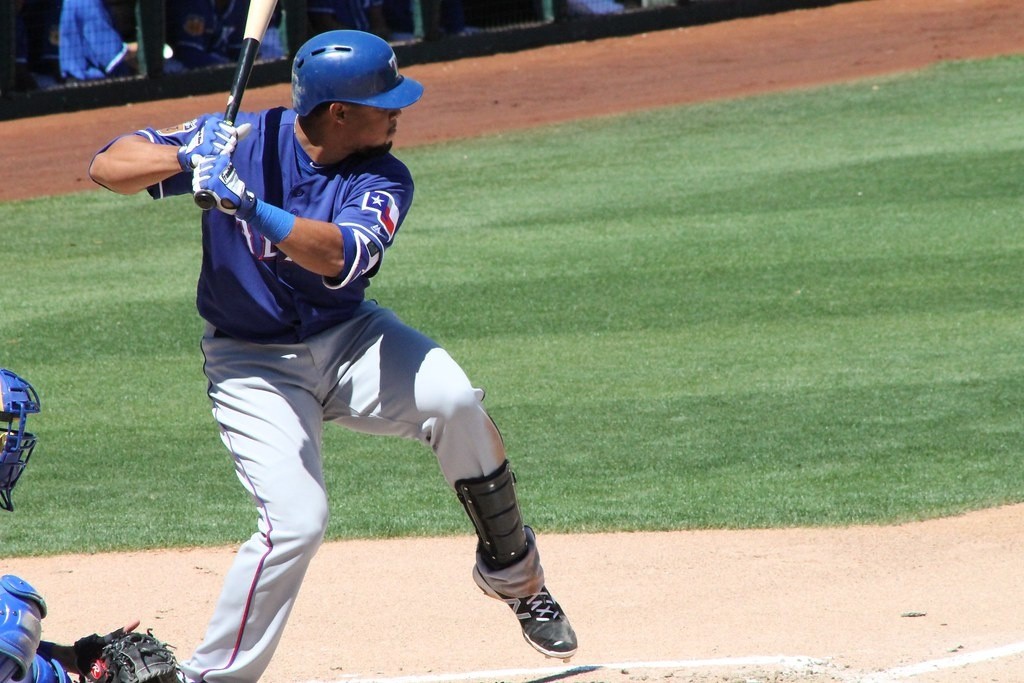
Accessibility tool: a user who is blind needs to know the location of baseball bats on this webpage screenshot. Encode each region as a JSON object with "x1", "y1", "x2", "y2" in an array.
[{"x1": 191, "y1": 0, "x2": 278, "y2": 212}]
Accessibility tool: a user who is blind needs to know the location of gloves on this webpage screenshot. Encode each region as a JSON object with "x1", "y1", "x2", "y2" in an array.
[
  {"x1": 191, "y1": 154, "x2": 256, "y2": 220},
  {"x1": 177, "y1": 117, "x2": 252, "y2": 172}
]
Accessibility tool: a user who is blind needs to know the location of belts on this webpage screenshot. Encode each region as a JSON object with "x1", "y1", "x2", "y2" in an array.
[{"x1": 213, "y1": 328, "x2": 233, "y2": 338}]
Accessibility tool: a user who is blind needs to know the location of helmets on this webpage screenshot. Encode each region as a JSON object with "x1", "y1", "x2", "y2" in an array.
[{"x1": 292, "y1": 29, "x2": 424, "y2": 116}]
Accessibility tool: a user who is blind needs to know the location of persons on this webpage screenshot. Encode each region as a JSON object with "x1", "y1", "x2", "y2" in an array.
[
  {"x1": 0, "y1": 369, "x2": 176, "y2": 683},
  {"x1": 88, "y1": 29, "x2": 578, "y2": 683},
  {"x1": 54, "y1": 0, "x2": 677, "y2": 84}
]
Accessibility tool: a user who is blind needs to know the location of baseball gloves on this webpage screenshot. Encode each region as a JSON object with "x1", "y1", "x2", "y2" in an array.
[{"x1": 77, "y1": 632, "x2": 180, "y2": 683}]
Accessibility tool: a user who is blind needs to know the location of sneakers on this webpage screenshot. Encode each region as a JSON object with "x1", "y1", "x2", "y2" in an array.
[{"x1": 473, "y1": 561, "x2": 578, "y2": 664}]
